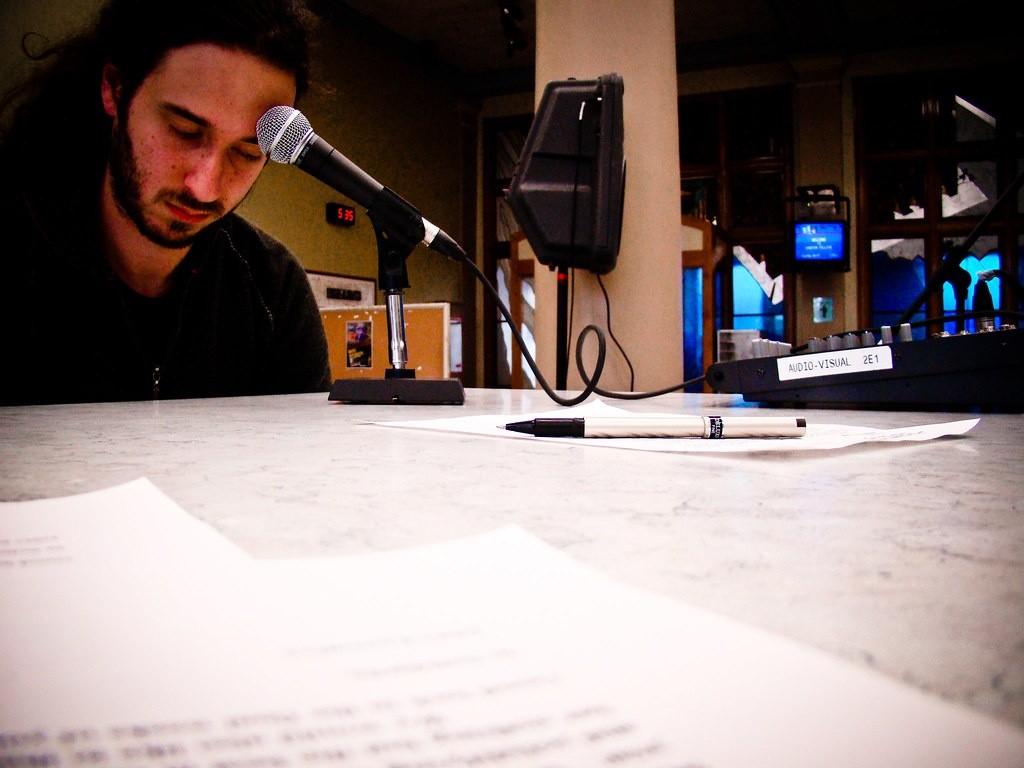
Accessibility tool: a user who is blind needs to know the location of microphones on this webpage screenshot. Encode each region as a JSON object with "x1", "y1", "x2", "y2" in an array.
[{"x1": 255, "y1": 105, "x2": 466, "y2": 261}]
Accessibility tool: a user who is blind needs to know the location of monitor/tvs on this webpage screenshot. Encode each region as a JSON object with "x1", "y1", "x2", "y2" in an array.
[{"x1": 792, "y1": 218, "x2": 850, "y2": 268}]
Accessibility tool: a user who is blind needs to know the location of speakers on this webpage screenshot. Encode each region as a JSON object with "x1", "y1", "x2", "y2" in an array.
[{"x1": 505, "y1": 73, "x2": 626, "y2": 276}]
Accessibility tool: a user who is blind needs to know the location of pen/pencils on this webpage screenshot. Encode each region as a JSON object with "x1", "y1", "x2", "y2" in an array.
[{"x1": 496, "y1": 416, "x2": 807, "y2": 439}]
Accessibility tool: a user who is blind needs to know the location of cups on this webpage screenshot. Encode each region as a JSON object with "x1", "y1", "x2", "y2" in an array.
[
  {"x1": 881, "y1": 326, "x2": 893, "y2": 344},
  {"x1": 901, "y1": 323, "x2": 912, "y2": 342},
  {"x1": 809, "y1": 330, "x2": 875, "y2": 353},
  {"x1": 752, "y1": 338, "x2": 792, "y2": 359}
]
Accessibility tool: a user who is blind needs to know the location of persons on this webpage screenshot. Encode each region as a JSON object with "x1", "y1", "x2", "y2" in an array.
[
  {"x1": 0, "y1": 1, "x2": 333, "y2": 406},
  {"x1": 348, "y1": 326, "x2": 371, "y2": 365}
]
